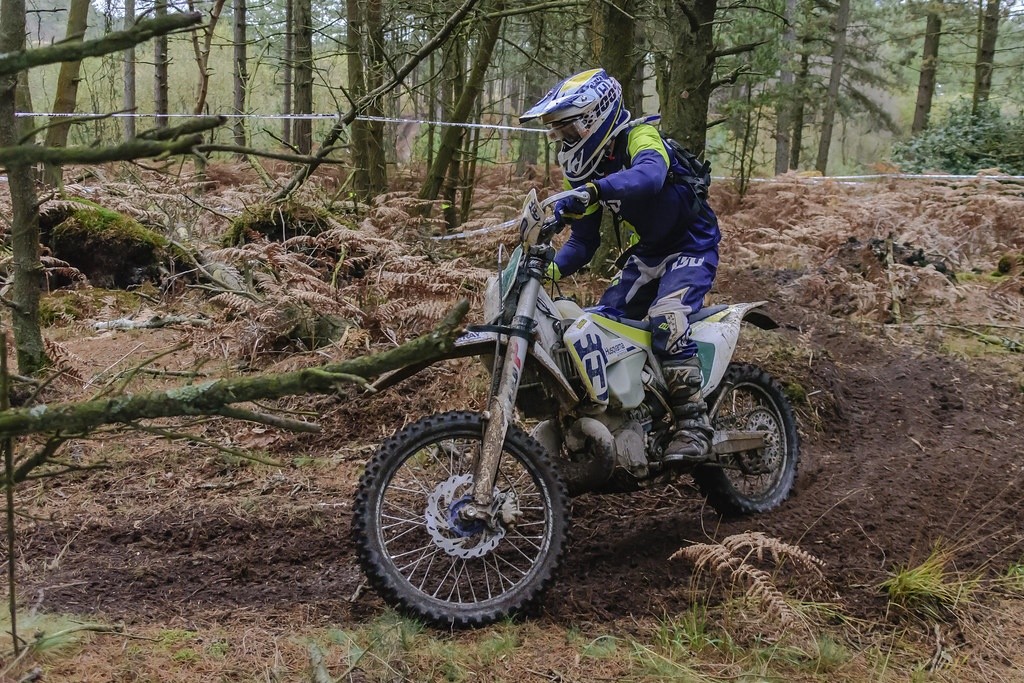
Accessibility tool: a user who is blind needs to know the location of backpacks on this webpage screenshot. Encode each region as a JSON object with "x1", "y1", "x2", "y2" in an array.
[{"x1": 613, "y1": 122, "x2": 712, "y2": 201}]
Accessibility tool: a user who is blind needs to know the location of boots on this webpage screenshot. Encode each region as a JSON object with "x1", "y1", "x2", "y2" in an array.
[{"x1": 662, "y1": 357, "x2": 717, "y2": 462}]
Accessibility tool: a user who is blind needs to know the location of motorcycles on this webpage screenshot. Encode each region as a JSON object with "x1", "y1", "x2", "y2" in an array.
[{"x1": 350, "y1": 187, "x2": 802, "y2": 632}]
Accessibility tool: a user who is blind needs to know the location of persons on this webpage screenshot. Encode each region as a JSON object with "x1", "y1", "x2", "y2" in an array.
[{"x1": 519, "y1": 68, "x2": 722, "y2": 465}]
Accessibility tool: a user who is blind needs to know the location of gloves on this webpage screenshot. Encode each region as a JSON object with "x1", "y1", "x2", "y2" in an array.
[{"x1": 554, "y1": 183, "x2": 600, "y2": 227}]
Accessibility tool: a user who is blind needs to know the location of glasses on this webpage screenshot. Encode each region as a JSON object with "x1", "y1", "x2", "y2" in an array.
[{"x1": 546, "y1": 121, "x2": 587, "y2": 142}]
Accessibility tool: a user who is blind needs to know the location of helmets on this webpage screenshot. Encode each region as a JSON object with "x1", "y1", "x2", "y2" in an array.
[{"x1": 519, "y1": 67, "x2": 631, "y2": 180}]
